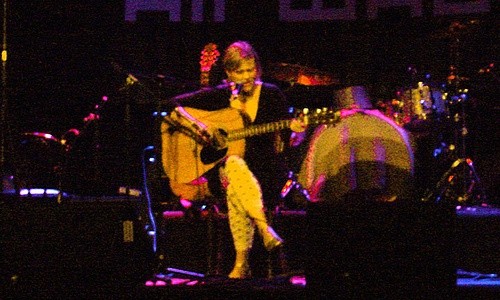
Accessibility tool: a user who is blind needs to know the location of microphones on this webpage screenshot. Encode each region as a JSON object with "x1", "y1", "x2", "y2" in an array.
[{"x1": 233, "y1": 84, "x2": 243, "y2": 99}]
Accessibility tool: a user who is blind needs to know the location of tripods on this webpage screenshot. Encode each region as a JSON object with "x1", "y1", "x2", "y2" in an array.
[{"x1": 420, "y1": 102, "x2": 485, "y2": 208}]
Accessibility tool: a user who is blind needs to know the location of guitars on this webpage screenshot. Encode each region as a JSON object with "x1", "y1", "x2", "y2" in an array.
[{"x1": 161, "y1": 106, "x2": 336, "y2": 184}]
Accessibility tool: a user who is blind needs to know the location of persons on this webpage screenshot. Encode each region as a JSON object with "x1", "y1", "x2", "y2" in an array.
[{"x1": 168, "y1": 41, "x2": 309, "y2": 279}]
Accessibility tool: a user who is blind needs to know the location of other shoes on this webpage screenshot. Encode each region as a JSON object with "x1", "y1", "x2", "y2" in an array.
[
  {"x1": 228, "y1": 261, "x2": 252, "y2": 279},
  {"x1": 263, "y1": 226, "x2": 284, "y2": 251}
]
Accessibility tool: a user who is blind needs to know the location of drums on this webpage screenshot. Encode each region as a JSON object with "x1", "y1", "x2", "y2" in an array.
[
  {"x1": 401, "y1": 87, "x2": 450, "y2": 129},
  {"x1": 303, "y1": 109, "x2": 415, "y2": 203}
]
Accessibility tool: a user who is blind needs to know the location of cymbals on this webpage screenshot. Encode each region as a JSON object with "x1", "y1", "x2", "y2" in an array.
[{"x1": 273, "y1": 63, "x2": 337, "y2": 86}]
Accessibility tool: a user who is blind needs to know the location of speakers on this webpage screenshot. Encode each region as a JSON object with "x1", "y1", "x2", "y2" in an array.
[
  {"x1": 0, "y1": 199, "x2": 141, "y2": 300},
  {"x1": 299, "y1": 202, "x2": 458, "y2": 300}
]
